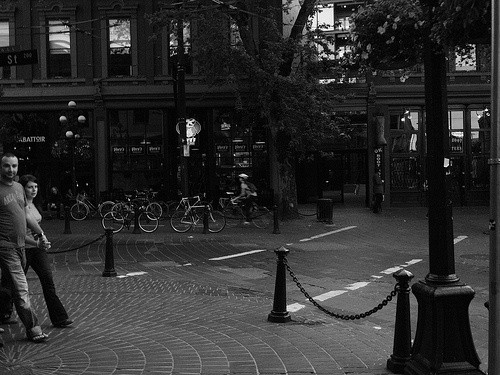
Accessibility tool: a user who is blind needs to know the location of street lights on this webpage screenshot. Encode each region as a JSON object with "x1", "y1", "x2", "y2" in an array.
[{"x1": 59, "y1": 100, "x2": 88, "y2": 195}]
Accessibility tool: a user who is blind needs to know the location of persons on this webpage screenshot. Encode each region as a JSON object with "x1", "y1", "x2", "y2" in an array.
[
  {"x1": 373, "y1": 167, "x2": 384, "y2": 214},
  {"x1": 455, "y1": 164, "x2": 471, "y2": 207},
  {"x1": 232, "y1": 173, "x2": 259, "y2": 226},
  {"x1": 0, "y1": 154, "x2": 73, "y2": 342}
]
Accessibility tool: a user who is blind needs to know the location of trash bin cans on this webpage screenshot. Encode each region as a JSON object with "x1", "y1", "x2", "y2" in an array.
[{"x1": 317, "y1": 199, "x2": 334, "y2": 224}]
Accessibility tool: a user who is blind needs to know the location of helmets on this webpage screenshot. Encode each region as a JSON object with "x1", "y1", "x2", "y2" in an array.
[{"x1": 239, "y1": 173, "x2": 249, "y2": 179}]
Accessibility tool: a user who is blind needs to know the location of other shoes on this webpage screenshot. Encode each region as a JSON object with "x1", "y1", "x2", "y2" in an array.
[
  {"x1": 242, "y1": 221, "x2": 250, "y2": 225},
  {"x1": 51, "y1": 320, "x2": 73, "y2": 326},
  {"x1": 26, "y1": 331, "x2": 49, "y2": 342},
  {"x1": 2, "y1": 314, "x2": 18, "y2": 324}
]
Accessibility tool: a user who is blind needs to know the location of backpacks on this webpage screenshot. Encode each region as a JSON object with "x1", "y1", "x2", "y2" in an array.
[{"x1": 240, "y1": 181, "x2": 258, "y2": 194}]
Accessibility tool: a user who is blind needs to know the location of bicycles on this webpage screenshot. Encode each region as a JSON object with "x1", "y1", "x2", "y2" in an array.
[
  {"x1": 164, "y1": 192, "x2": 227, "y2": 234},
  {"x1": 216, "y1": 192, "x2": 274, "y2": 229},
  {"x1": 69, "y1": 189, "x2": 169, "y2": 233}
]
